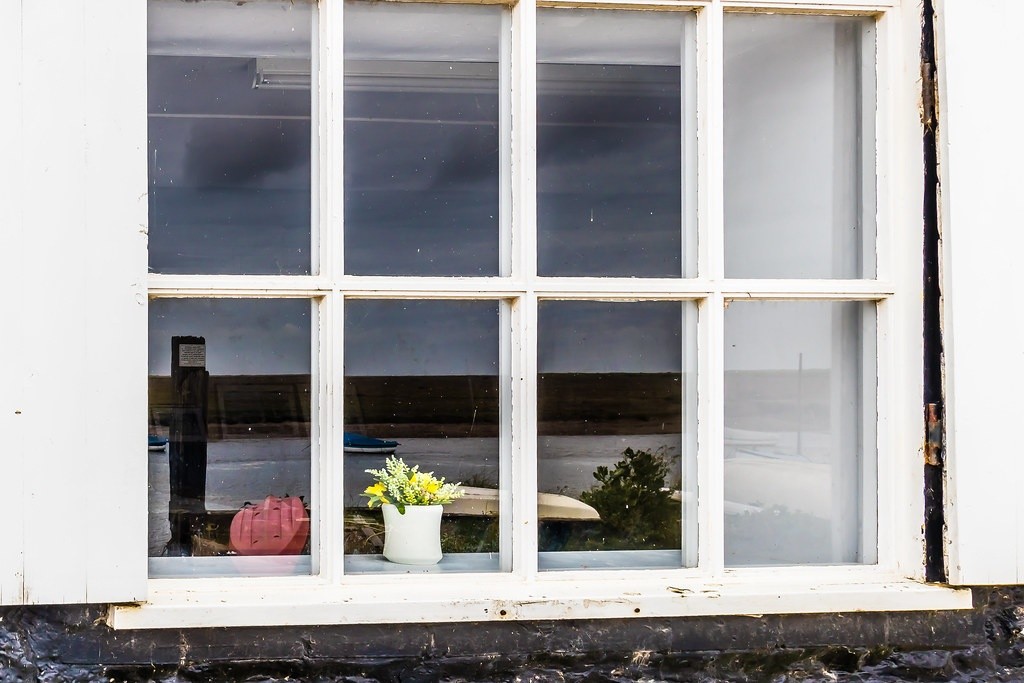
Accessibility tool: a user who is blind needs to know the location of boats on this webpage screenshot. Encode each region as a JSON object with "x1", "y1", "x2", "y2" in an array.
[
  {"x1": 629, "y1": 483, "x2": 682, "y2": 501},
  {"x1": 344, "y1": 432, "x2": 402, "y2": 454},
  {"x1": 148, "y1": 433, "x2": 170, "y2": 451},
  {"x1": 444, "y1": 485, "x2": 603, "y2": 524},
  {"x1": 228, "y1": 493, "x2": 309, "y2": 556}
]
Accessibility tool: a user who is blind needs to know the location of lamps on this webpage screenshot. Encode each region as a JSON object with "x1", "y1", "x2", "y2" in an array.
[{"x1": 244, "y1": 59, "x2": 680, "y2": 92}]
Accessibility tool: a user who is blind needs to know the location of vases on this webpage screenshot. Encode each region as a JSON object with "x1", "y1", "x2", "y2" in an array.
[{"x1": 378, "y1": 503, "x2": 446, "y2": 564}]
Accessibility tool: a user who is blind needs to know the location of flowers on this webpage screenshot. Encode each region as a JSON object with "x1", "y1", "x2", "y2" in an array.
[{"x1": 360, "y1": 456, "x2": 467, "y2": 508}]
